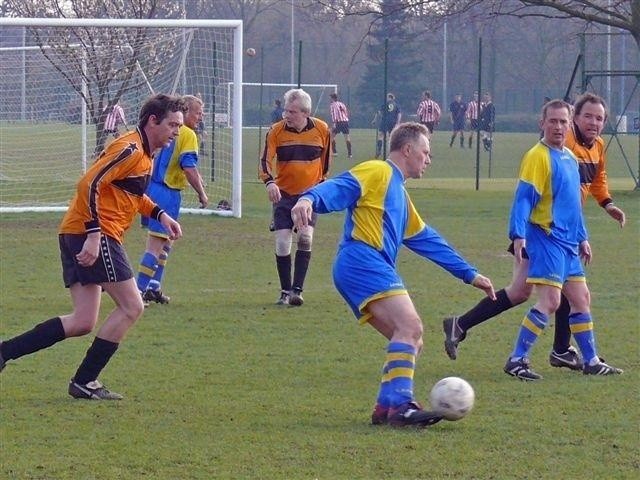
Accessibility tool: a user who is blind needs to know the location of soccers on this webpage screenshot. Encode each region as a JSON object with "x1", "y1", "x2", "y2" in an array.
[
  {"x1": 247, "y1": 48, "x2": 255, "y2": 56},
  {"x1": 430, "y1": 377, "x2": 476, "y2": 421}
]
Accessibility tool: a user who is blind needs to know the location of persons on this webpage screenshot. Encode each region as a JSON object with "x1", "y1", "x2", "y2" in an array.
[
  {"x1": 443, "y1": 92, "x2": 626, "y2": 371},
  {"x1": 272, "y1": 100, "x2": 283, "y2": 124},
  {"x1": 509, "y1": 99, "x2": 623, "y2": 381},
  {"x1": 325, "y1": 93, "x2": 353, "y2": 157},
  {"x1": 0, "y1": 96, "x2": 182, "y2": 399},
  {"x1": 539, "y1": 97, "x2": 574, "y2": 140},
  {"x1": 93, "y1": 91, "x2": 205, "y2": 158},
  {"x1": 259, "y1": 89, "x2": 332, "y2": 307},
  {"x1": 292, "y1": 122, "x2": 497, "y2": 426},
  {"x1": 374, "y1": 93, "x2": 495, "y2": 159},
  {"x1": 135, "y1": 95, "x2": 209, "y2": 307}
]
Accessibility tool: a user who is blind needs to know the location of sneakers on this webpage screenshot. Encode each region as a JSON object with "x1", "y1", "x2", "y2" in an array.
[
  {"x1": 504, "y1": 357, "x2": 544, "y2": 381},
  {"x1": 290, "y1": 287, "x2": 303, "y2": 305},
  {"x1": 347, "y1": 155, "x2": 352, "y2": 158},
  {"x1": 140, "y1": 289, "x2": 170, "y2": 307},
  {"x1": 443, "y1": 317, "x2": 467, "y2": 360},
  {"x1": 372, "y1": 400, "x2": 444, "y2": 426},
  {"x1": 68, "y1": 377, "x2": 124, "y2": 400},
  {"x1": 582, "y1": 356, "x2": 623, "y2": 376},
  {"x1": 549, "y1": 346, "x2": 584, "y2": 370},
  {"x1": 275, "y1": 290, "x2": 290, "y2": 304},
  {"x1": 333, "y1": 152, "x2": 338, "y2": 156}
]
